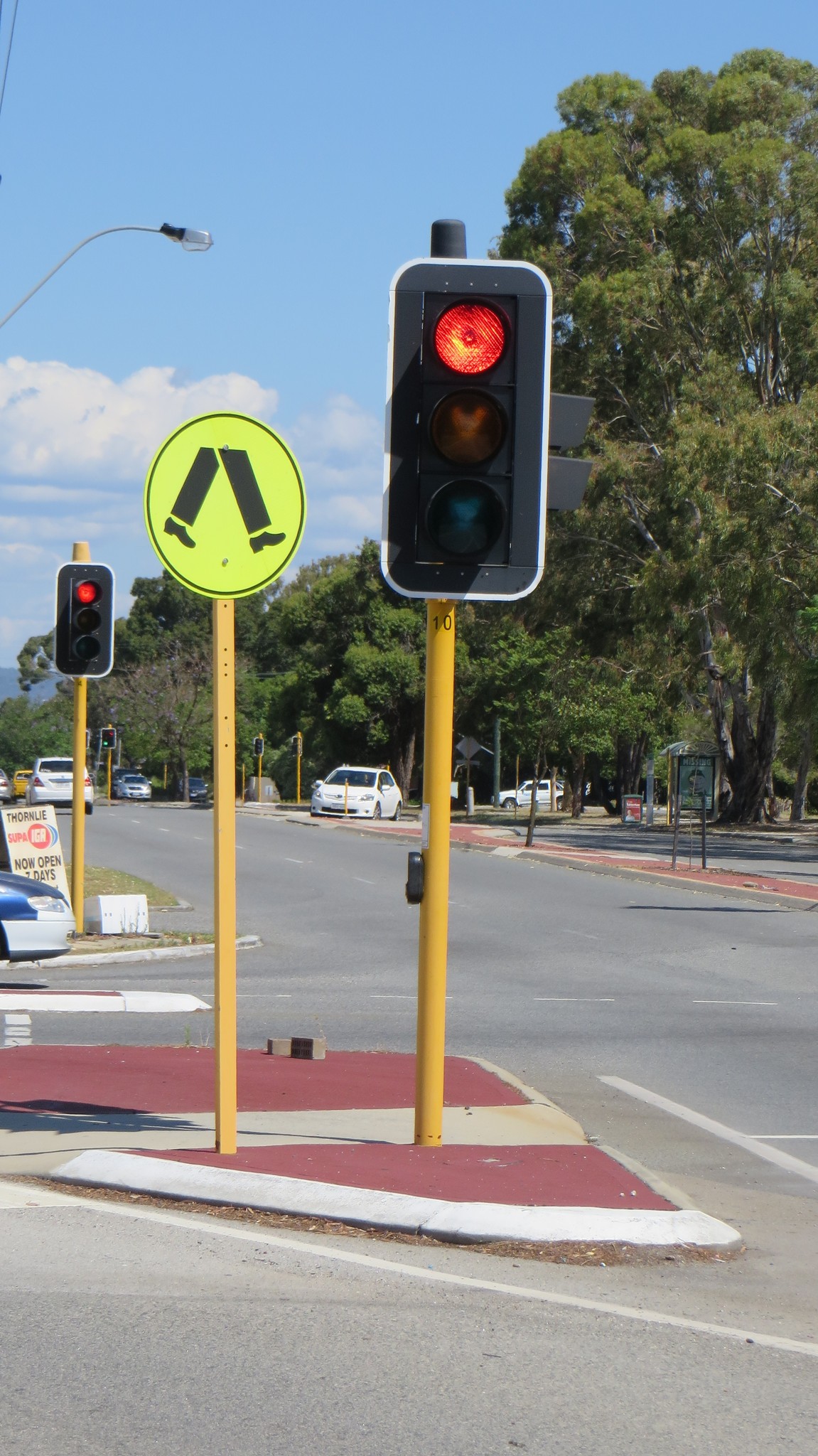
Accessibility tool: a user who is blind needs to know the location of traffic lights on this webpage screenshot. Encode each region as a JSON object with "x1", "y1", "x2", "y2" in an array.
[
  {"x1": 256, "y1": 739, "x2": 262, "y2": 753},
  {"x1": 103, "y1": 731, "x2": 115, "y2": 746},
  {"x1": 54, "y1": 562, "x2": 114, "y2": 676},
  {"x1": 389, "y1": 257, "x2": 550, "y2": 602}
]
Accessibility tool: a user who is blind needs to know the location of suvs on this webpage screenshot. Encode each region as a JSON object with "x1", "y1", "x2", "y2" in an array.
[{"x1": 112, "y1": 769, "x2": 142, "y2": 796}]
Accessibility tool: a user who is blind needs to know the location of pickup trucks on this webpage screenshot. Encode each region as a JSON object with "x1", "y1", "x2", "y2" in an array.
[{"x1": 491, "y1": 780, "x2": 564, "y2": 811}]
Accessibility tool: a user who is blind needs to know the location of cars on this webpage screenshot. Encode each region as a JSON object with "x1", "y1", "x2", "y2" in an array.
[
  {"x1": 0, "y1": 768, "x2": 12, "y2": 804},
  {"x1": 13, "y1": 769, "x2": 33, "y2": 801},
  {"x1": 117, "y1": 775, "x2": 152, "y2": 800},
  {"x1": 0, "y1": 869, "x2": 72, "y2": 965},
  {"x1": 23, "y1": 757, "x2": 95, "y2": 815},
  {"x1": 178, "y1": 778, "x2": 210, "y2": 802},
  {"x1": 310, "y1": 767, "x2": 403, "y2": 821}
]
